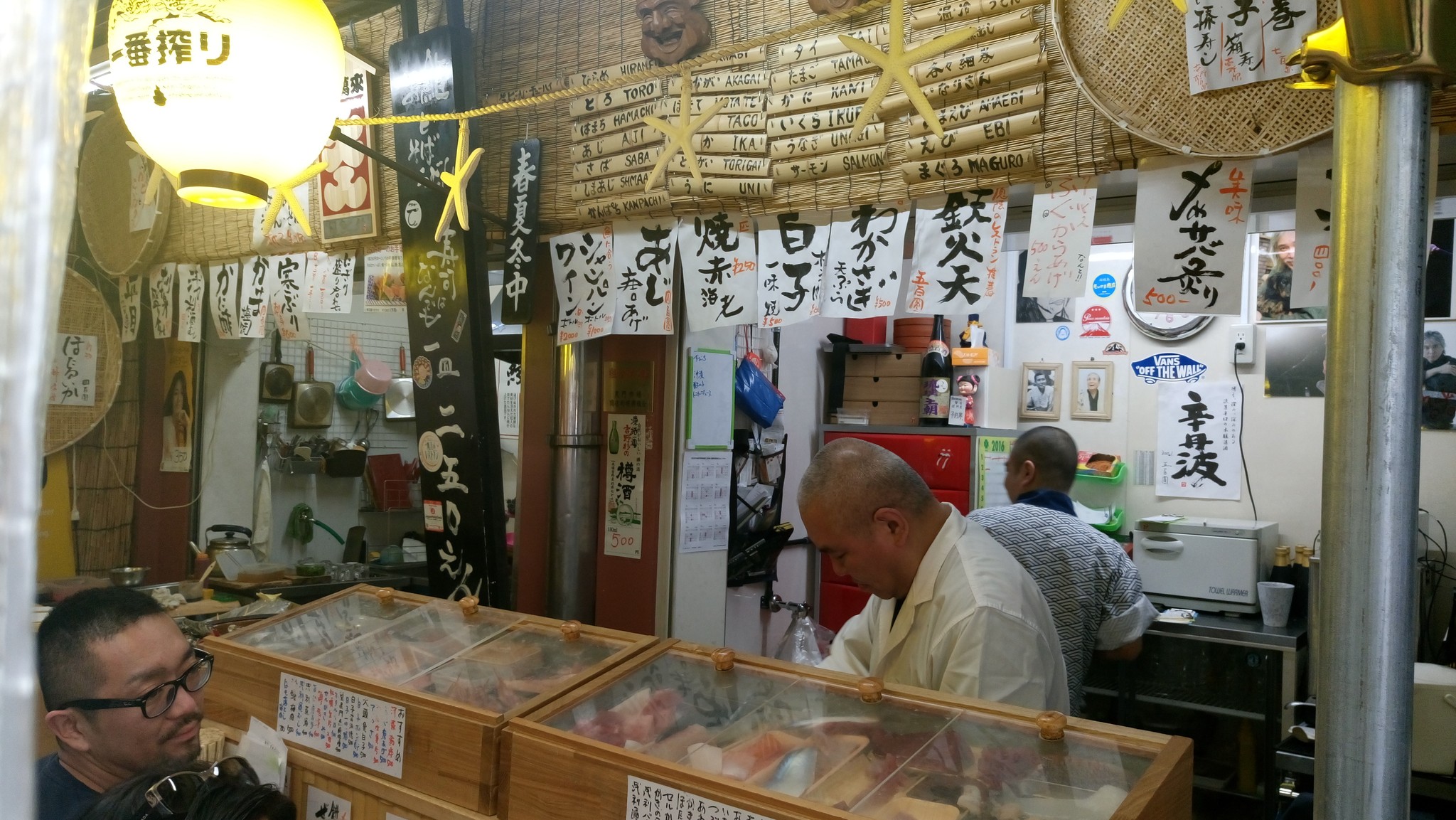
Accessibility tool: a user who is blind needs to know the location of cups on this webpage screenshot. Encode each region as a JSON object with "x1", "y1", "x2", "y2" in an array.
[
  {"x1": 330, "y1": 563, "x2": 370, "y2": 580},
  {"x1": 688, "y1": 742, "x2": 721, "y2": 776},
  {"x1": 1257, "y1": 582, "x2": 1295, "y2": 627}
]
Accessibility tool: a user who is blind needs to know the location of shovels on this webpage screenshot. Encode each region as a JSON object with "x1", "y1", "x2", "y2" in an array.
[{"x1": 294, "y1": 447, "x2": 312, "y2": 461}]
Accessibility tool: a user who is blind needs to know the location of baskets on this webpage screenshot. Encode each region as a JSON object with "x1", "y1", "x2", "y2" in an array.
[
  {"x1": 77, "y1": 102, "x2": 172, "y2": 275},
  {"x1": 45, "y1": 266, "x2": 123, "y2": 454}
]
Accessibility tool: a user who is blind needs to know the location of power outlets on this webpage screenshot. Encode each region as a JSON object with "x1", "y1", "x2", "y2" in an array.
[{"x1": 1229, "y1": 324, "x2": 1256, "y2": 364}]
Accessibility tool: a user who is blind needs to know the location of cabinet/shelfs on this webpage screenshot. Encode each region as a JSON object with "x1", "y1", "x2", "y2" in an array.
[
  {"x1": 1075, "y1": 463, "x2": 1126, "y2": 532},
  {"x1": 1082, "y1": 605, "x2": 1311, "y2": 820}
]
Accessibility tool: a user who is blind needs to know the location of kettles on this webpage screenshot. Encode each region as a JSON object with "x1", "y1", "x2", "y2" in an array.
[{"x1": 189, "y1": 524, "x2": 254, "y2": 577}]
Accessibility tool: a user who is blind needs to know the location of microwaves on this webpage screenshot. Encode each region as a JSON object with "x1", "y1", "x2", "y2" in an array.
[{"x1": 1132, "y1": 517, "x2": 1277, "y2": 618}]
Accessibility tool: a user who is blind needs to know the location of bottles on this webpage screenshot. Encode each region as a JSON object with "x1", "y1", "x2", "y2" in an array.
[
  {"x1": 1269, "y1": 544, "x2": 1314, "y2": 622},
  {"x1": 194, "y1": 550, "x2": 209, "y2": 588},
  {"x1": 917, "y1": 315, "x2": 952, "y2": 426}
]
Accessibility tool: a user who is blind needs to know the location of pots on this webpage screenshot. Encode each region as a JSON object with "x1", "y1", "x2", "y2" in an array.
[
  {"x1": 385, "y1": 343, "x2": 417, "y2": 420},
  {"x1": 260, "y1": 328, "x2": 295, "y2": 401},
  {"x1": 287, "y1": 341, "x2": 338, "y2": 429}
]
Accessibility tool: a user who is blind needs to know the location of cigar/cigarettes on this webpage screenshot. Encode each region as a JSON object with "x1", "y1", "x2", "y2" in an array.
[{"x1": 1043, "y1": 385, "x2": 1045, "y2": 386}]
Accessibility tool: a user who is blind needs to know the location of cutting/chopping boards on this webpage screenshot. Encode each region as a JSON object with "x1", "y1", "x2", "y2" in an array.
[{"x1": 363, "y1": 454, "x2": 412, "y2": 510}]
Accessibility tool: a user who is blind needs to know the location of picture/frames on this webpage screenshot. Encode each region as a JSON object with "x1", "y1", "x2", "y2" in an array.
[
  {"x1": 1018, "y1": 362, "x2": 1063, "y2": 419},
  {"x1": 1072, "y1": 361, "x2": 1113, "y2": 419}
]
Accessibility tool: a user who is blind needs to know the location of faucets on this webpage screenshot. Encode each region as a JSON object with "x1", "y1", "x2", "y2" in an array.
[{"x1": 299, "y1": 506, "x2": 318, "y2": 524}]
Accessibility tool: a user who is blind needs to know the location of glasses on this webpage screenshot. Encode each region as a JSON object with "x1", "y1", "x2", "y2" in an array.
[
  {"x1": 131, "y1": 755, "x2": 261, "y2": 820},
  {"x1": 57, "y1": 647, "x2": 214, "y2": 719}
]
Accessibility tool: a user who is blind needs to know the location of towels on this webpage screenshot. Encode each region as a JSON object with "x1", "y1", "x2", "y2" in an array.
[
  {"x1": 251, "y1": 456, "x2": 272, "y2": 561},
  {"x1": 286, "y1": 503, "x2": 314, "y2": 544}
]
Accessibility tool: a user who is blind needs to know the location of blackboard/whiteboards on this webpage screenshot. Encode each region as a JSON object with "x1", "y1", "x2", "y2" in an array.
[{"x1": 686, "y1": 346, "x2": 736, "y2": 450}]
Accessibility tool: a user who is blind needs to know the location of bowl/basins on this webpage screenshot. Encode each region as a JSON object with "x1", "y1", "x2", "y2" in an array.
[
  {"x1": 296, "y1": 561, "x2": 331, "y2": 576},
  {"x1": 237, "y1": 562, "x2": 285, "y2": 585},
  {"x1": 106, "y1": 566, "x2": 151, "y2": 587},
  {"x1": 50, "y1": 576, "x2": 103, "y2": 600},
  {"x1": 177, "y1": 579, "x2": 204, "y2": 603}
]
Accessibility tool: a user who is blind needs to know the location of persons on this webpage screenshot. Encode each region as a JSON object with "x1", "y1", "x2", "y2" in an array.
[
  {"x1": 965, "y1": 426, "x2": 1160, "y2": 716},
  {"x1": 956, "y1": 374, "x2": 980, "y2": 427},
  {"x1": 163, "y1": 370, "x2": 191, "y2": 446},
  {"x1": 1257, "y1": 230, "x2": 1328, "y2": 319},
  {"x1": 34, "y1": 585, "x2": 214, "y2": 820},
  {"x1": 81, "y1": 756, "x2": 297, "y2": 820},
  {"x1": 1076, "y1": 373, "x2": 1104, "y2": 412},
  {"x1": 1421, "y1": 330, "x2": 1456, "y2": 429},
  {"x1": 1027, "y1": 373, "x2": 1055, "y2": 413},
  {"x1": 704, "y1": 438, "x2": 1070, "y2": 778}
]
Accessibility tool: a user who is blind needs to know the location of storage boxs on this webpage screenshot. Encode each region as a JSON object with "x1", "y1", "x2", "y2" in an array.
[
  {"x1": 842, "y1": 352, "x2": 924, "y2": 427},
  {"x1": 951, "y1": 348, "x2": 998, "y2": 366}
]
